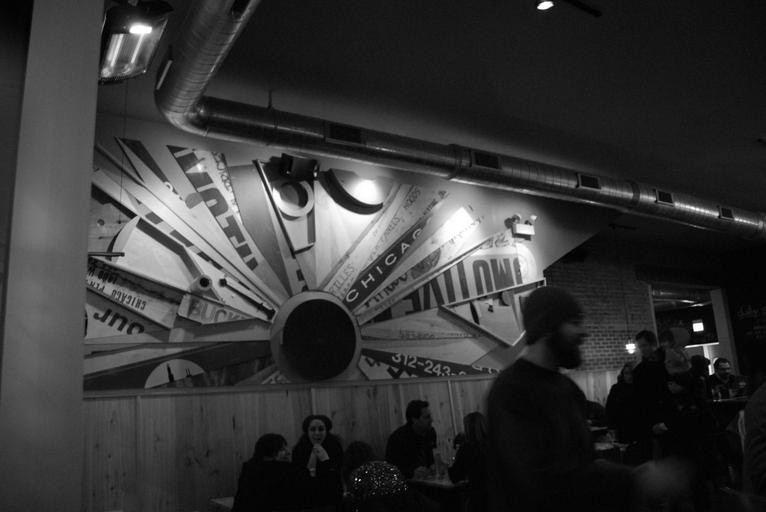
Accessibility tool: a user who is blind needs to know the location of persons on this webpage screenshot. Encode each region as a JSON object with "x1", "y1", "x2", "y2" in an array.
[
  {"x1": 384, "y1": 399, "x2": 438, "y2": 479},
  {"x1": 291, "y1": 414, "x2": 344, "y2": 511},
  {"x1": 482, "y1": 286, "x2": 673, "y2": 512},
  {"x1": 448, "y1": 411, "x2": 485, "y2": 483},
  {"x1": 583, "y1": 327, "x2": 766, "y2": 511},
  {"x1": 229, "y1": 431, "x2": 307, "y2": 511}
]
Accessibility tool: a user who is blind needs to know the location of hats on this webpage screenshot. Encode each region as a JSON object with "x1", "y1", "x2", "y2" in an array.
[
  {"x1": 339, "y1": 459, "x2": 410, "y2": 502},
  {"x1": 517, "y1": 285, "x2": 585, "y2": 345}
]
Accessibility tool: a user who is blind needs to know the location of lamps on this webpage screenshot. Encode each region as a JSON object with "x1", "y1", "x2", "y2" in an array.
[
  {"x1": 512, "y1": 213, "x2": 537, "y2": 236},
  {"x1": 99, "y1": 0, "x2": 175, "y2": 86}
]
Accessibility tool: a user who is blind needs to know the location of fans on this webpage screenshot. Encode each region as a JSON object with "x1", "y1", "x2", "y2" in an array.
[{"x1": 407, "y1": 473, "x2": 469, "y2": 489}]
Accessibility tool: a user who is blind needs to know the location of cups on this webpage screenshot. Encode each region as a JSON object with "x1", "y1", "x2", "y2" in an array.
[
  {"x1": 431, "y1": 447, "x2": 446, "y2": 483},
  {"x1": 712, "y1": 389, "x2": 722, "y2": 403}
]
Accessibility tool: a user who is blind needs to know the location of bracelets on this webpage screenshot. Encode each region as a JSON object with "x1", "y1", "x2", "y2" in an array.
[{"x1": 308, "y1": 466, "x2": 316, "y2": 473}]
